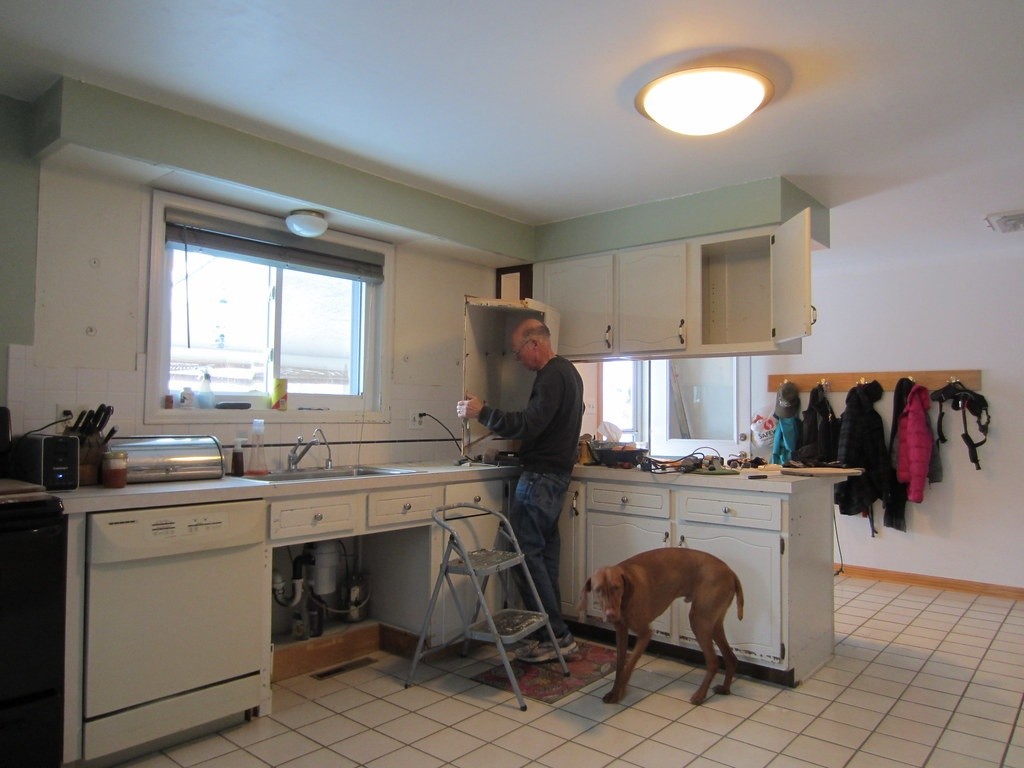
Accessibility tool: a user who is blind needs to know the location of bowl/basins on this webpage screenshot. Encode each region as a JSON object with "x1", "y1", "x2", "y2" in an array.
[{"x1": 586, "y1": 439, "x2": 648, "y2": 468}]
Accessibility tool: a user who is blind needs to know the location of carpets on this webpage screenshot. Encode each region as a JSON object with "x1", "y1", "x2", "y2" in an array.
[{"x1": 469, "y1": 641, "x2": 632, "y2": 704}]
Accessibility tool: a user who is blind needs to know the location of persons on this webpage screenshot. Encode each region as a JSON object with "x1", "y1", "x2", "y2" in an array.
[{"x1": 457, "y1": 318, "x2": 586, "y2": 663}]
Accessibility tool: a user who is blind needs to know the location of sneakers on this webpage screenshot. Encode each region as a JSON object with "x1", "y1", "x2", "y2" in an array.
[{"x1": 515, "y1": 629, "x2": 579, "y2": 662}]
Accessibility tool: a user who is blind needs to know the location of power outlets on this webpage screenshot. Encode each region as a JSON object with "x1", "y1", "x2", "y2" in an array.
[
  {"x1": 57, "y1": 402, "x2": 76, "y2": 432},
  {"x1": 409, "y1": 409, "x2": 425, "y2": 430}
]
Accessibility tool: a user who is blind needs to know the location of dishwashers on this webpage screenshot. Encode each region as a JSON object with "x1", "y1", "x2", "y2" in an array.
[{"x1": 84, "y1": 498, "x2": 268, "y2": 766}]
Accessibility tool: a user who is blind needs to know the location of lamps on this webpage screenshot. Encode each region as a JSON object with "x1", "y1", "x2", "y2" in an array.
[
  {"x1": 285, "y1": 210, "x2": 329, "y2": 237},
  {"x1": 634, "y1": 65, "x2": 774, "y2": 136}
]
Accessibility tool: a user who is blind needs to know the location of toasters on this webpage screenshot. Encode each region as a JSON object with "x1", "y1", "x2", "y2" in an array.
[{"x1": 14, "y1": 433, "x2": 81, "y2": 493}]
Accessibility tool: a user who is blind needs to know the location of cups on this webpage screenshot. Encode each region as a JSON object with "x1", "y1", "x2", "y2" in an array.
[{"x1": 577, "y1": 441, "x2": 595, "y2": 466}]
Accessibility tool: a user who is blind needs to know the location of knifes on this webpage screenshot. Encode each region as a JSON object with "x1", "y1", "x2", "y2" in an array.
[{"x1": 71, "y1": 403, "x2": 117, "y2": 447}]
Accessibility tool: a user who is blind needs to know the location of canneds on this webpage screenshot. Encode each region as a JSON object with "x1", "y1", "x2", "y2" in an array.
[
  {"x1": 102, "y1": 452, "x2": 127, "y2": 489},
  {"x1": 272, "y1": 378, "x2": 288, "y2": 410}
]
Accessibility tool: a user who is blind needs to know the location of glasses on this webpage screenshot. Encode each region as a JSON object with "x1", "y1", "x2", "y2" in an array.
[{"x1": 511, "y1": 340, "x2": 529, "y2": 361}]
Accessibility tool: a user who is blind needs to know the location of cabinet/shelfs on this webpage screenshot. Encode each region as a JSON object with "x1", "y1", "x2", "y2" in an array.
[
  {"x1": 545, "y1": 206, "x2": 816, "y2": 362},
  {"x1": 557, "y1": 477, "x2": 846, "y2": 687},
  {"x1": 271, "y1": 479, "x2": 512, "y2": 659}
]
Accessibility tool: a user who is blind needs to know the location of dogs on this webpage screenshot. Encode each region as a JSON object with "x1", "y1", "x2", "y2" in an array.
[{"x1": 580, "y1": 547, "x2": 745, "y2": 706}]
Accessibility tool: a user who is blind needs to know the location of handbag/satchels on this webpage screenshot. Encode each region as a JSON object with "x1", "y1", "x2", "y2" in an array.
[{"x1": 751, "y1": 411, "x2": 779, "y2": 448}]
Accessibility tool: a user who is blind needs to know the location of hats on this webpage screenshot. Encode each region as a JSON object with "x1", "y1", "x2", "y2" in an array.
[{"x1": 774, "y1": 382, "x2": 798, "y2": 418}]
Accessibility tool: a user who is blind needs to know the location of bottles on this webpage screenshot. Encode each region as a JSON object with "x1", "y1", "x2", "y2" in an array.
[
  {"x1": 101, "y1": 452, "x2": 128, "y2": 487},
  {"x1": 246, "y1": 418, "x2": 268, "y2": 475},
  {"x1": 163, "y1": 373, "x2": 215, "y2": 410}
]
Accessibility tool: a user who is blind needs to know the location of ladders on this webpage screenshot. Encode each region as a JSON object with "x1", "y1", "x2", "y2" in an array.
[{"x1": 402, "y1": 503, "x2": 570, "y2": 711}]
